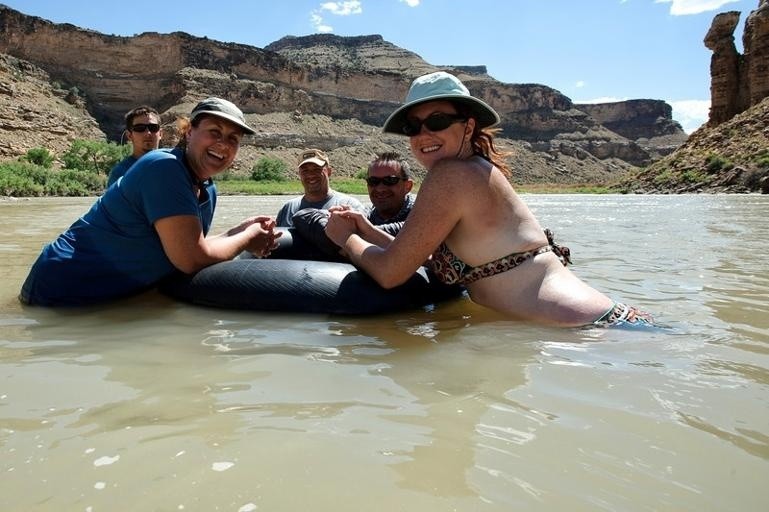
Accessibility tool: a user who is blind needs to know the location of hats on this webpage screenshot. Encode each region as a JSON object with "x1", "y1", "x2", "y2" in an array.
[
  {"x1": 190, "y1": 97, "x2": 256, "y2": 135},
  {"x1": 382, "y1": 72, "x2": 501, "y2": 135},
  {"x1": 298, "y1": 149, "x2": 329, "y2": 168}
]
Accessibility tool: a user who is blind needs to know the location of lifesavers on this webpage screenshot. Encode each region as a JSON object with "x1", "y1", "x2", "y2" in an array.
[{"x1": 167, "y1": 227, "x2": 464, "y2": 317}]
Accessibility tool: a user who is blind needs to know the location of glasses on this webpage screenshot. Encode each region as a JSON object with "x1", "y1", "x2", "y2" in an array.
[
  {"x1": 366, "y1": 177, "x2": 400, "y2": 187},
  {"x1": 130, "y1": 123, "x2": 160, "y2": 133},
  {"x1": 400, "y1": 111, "x2": 466, "y2": 136}
]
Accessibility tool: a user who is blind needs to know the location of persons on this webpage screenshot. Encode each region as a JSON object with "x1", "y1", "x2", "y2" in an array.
[
  {"x1": 107, "y1": 106, "x2": 161, "y2": 188},
  {"x1": 17, "y1": 98, "x2": 283, "y2": 307},
  {"x1": 324, "y1": 71, "x2": 661, "y2": 330},
  {"x1": 357, "y1": 151, "x2": 419, "y2": 237},
  {"x1": 276, "y1": 149, "x2": 363, "y2": 227}
]
